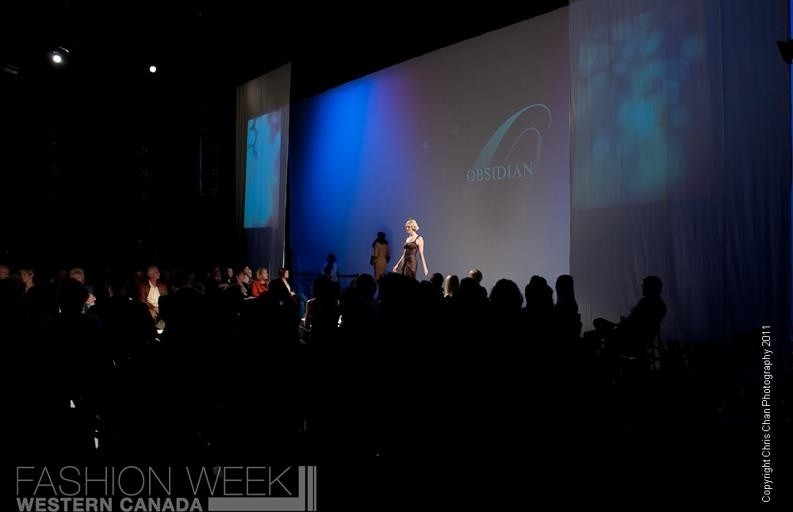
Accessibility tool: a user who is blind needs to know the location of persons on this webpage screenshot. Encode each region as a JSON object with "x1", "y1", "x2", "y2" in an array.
[
  {"x1": 371, "y1": 231, "x2": 390, "y2": 278},
  {"x1": 319, "y1": 254, "x2": 338, "y2": 282},
  {"x1": 0, "y1": 263, "x2": 665, "y2": 512},
  {"x1": 393, "y1": 219, "x2": 429, "y2": 278}
]
